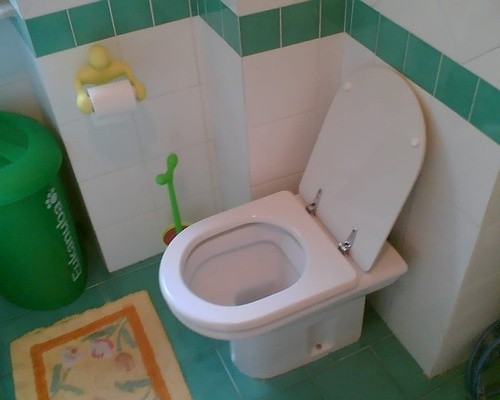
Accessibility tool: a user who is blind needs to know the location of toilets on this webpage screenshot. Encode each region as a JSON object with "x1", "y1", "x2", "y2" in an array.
[{"x1": 158, "y1": 68, "x2": 429, "y2": 380}]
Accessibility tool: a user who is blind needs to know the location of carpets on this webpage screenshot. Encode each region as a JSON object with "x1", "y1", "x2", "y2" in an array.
[{"x1": 10, "y1": 290, "x2": 193, "y2": 400}]
